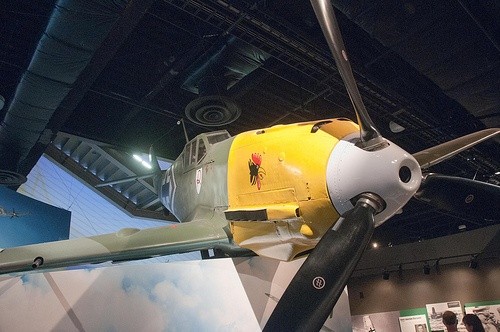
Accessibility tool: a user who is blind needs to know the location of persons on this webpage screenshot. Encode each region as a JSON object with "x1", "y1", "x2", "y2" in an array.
[
  {"x1": 442, "y1": 311, "x2": 460, "y2": 332},
  {"x1": 463, "y1": 313, "x2": 485, "y2": 332}
]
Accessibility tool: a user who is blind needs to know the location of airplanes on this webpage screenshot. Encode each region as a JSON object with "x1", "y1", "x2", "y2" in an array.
[{"x1": 0, "y1": 0, "x2": 499, "y2": 331}]
